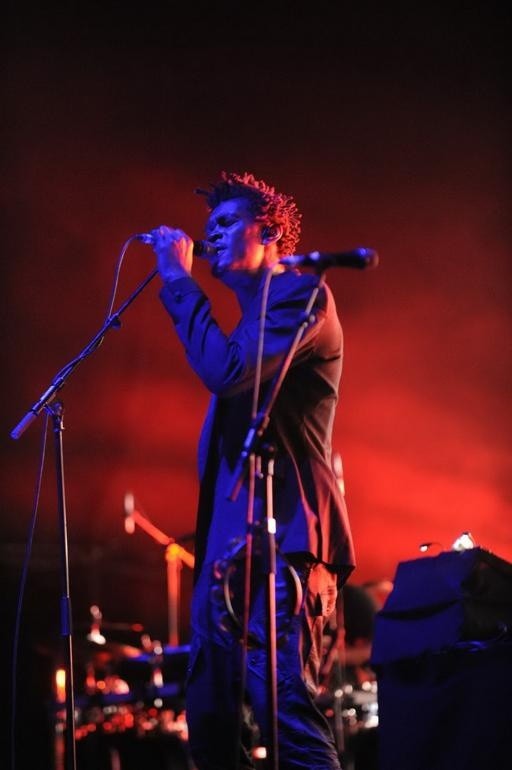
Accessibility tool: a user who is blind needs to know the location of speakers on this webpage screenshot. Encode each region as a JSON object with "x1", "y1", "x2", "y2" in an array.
[{"x1": 373, "y1": 553, "x2": 512, "y2": 770}]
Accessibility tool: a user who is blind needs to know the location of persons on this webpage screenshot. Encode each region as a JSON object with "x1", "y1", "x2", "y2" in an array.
[{"x1": 147, "y1": 169, "x2": 346, "y2": 769}]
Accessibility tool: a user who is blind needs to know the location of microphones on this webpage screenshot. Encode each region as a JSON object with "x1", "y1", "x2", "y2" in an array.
[
  {"x1": 124, "y1": 484, "x2": 138, "y2": 533},
  {"x1": 282, "y1": 245, "x2": 380, "y2": 272},
  {"x1": 137, "y1": 231, "x2": 215, "y2": 258}
]
